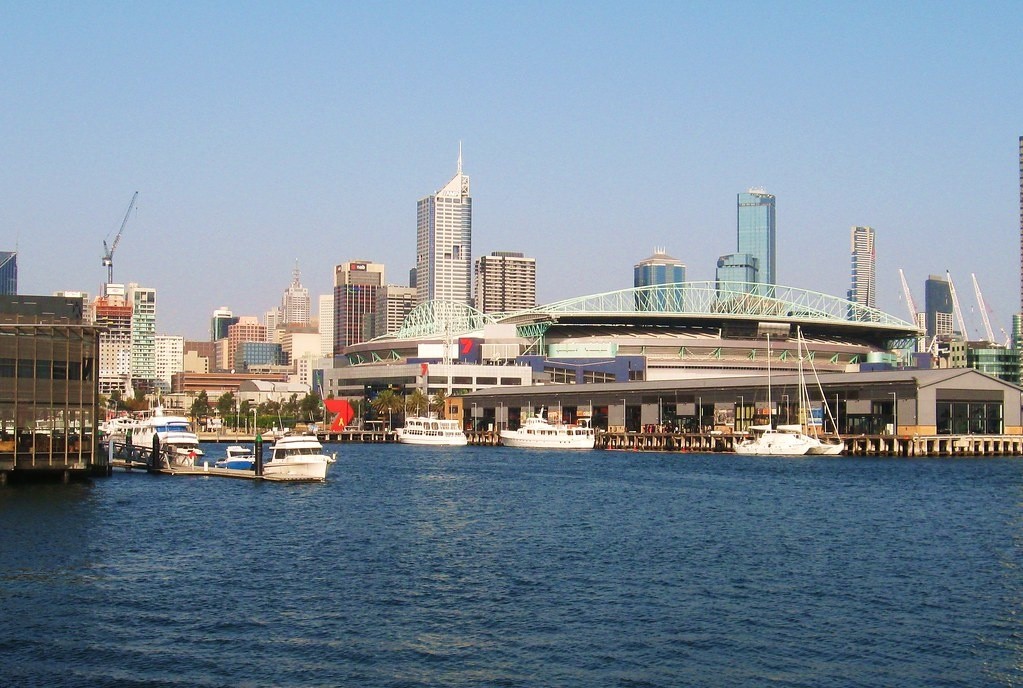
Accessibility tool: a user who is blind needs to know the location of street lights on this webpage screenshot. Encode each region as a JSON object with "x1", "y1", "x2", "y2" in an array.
[
  {"x1": 888, "y1": 391, "x2": 896, "y2": 436},
  {"x1": 696, "y1": 397, "x2": 701, "y2": 434},
  {"x1": 781, "y1": 394, "x2": 789, "y2": 425},
  {"x1": 472, "y1": 402, "x2": 476, "y2": 431},
  {"x1": 737, "y1": 395, "x2": 744, "y2": 431},
  {"x1": 620, "y1": 399, "x2": 626, "y2": 433},
  {"x1": 498, "y1": 401, "x2": 503, "y2": 429},
  {"x1": 832, "y1": 393, "x2": 839, "y2": 436},
  {"x1": 388, "y1": 407, "x2": 392, "y2": 432}
]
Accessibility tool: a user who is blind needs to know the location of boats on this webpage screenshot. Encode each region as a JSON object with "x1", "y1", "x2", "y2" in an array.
[
  {"x1": 262, "y1": 426, "x2": 335, "y2": 482},
  {"x1": 214, "y1": 446, "x2": 256, "y2": 470},
  {"x1": 498, "y1": 413, "x2": 596, "y2": 450},
  {"x1": 395, "y1": 416, "x2": 468, "y2": 446},
  {"x1": 100, "y1": 403, "x2": 205, "y2": 467}
]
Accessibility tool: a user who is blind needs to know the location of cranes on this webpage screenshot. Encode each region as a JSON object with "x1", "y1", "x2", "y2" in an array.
[
  {"x1": 100, "y1": 189, "x2": 139, "y2": 285},
  {"x1": 971, "y1": 271, "x2": 997, "y2": 349},
  {"x1": 898, "y1": 267, "x2": 922, "y2": 329},
  {"x1": 946, "y1": 268, "x2": 969, "y2": 341}
]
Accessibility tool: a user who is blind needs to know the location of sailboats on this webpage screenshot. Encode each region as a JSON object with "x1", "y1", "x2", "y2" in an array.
[
  {"x1": 731, "y1": 332, "x2": 812, "y2": 456},
  {"x1": 773, "y1": 323, "x2": 845, "y2": 455}
]
